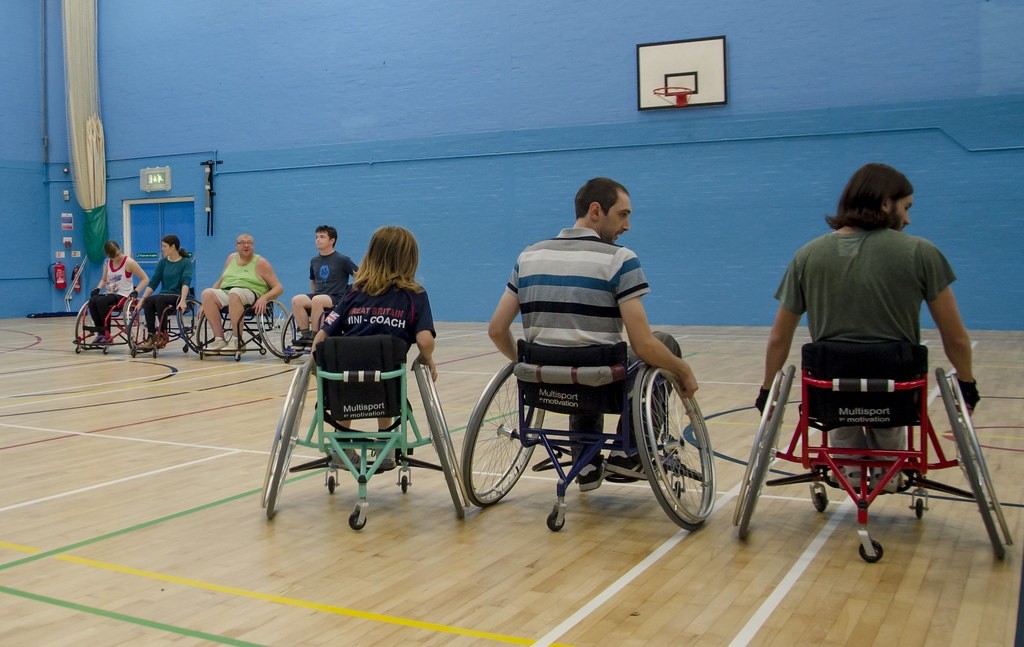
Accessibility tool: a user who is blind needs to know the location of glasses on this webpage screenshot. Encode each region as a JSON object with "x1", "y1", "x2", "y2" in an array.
[{"x1": 237, "y1": 241, "x2": 254, "y2": 245}]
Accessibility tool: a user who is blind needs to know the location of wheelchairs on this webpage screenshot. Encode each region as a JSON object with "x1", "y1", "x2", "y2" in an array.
[
  {"x1": 127, "y1": 287, "x2": 208, "y2": 358},
  {"x1": 260, "y1": 333, "x2": 471, "y2": 522},
  {"x1": 71, "y1": 290, "x2": 149, "y2": 357},
  {"x1": 731, "y1": 338, "x2": 1016, "y2": 564},
  {"x1": 281, "y1": 282, "x2": 354, "y2": 364},
  {"x1": 459, "y1": 338, "x2": 716, "y2": 533},
  {"x1": 195, "y1": 296, "x2": 295, "y2": 360}
]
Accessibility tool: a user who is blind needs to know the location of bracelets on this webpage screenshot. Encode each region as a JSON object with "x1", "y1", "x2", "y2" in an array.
[{"x1": 142, "y1": 296, "x2": 147, "y2": 301}]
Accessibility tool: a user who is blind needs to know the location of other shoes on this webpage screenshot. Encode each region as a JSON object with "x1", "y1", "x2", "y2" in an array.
[
  {"x1": 203, "y1": 340, "x2": 227, "y2": 350},
  {"x1": 331, "y1": 450, "x2": 361, "y2": 469},
  {"x1": 292, "y1": 336, "x2": 314, "y2": 346},
  {"x1": 829, "y1": 466, "x2": 862, "y2": 487},
  {"x1": 371, "y1": 440, "x2": 396, "y2": 470},
  {"x1": 156, "y1": 332, "x2": 169, "y2": 348},
  {"x1": 604, "y1": 448, "x2": 660, "y2": 480},
  {"x1": 137, "y1": 332, "x2": 157, "y2": 349},
  {"x1": 219, "y1": 339, "x2": 247, "y2": 354},
  {"x1": 93, "y1": 335, "x2": 113, "y2": 343},
  {"x1": 576, "y1": 454, "x2": 603, "y2": 492},
  {"x1": 869, "y1": 471, "x2": 901, "y2": 494}
]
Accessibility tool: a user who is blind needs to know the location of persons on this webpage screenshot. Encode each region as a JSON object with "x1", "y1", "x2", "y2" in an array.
[
  {"x1": 134, "y1": 234, "x2": 194, "y2": 352},
  {"x1": 291, "y1": 226, "x2": 358, "y2": 351},
  {"x1": 754, "y1": 163, "x2": 981, "y2": 494},
  {"x1": 486, "y1": 178, "x2": 700, "y2": 492},
  {"x1": 88, "y1": 240, "x2": 150, "y2": 344},
  {"x1": 301, "y1": 225, "x2": 439, "y2": 472},
  {"x1": 196, "y1": 234, "x2": 285, "y2": 357}
]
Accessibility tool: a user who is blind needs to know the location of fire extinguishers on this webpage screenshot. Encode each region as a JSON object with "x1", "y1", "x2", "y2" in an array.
[
  {"x1": 73, "y1": 264, "x2": 81, "y2": 294},
  {"x1": 48, "y1": 261, "x2": 67, "y2": 289}
]
